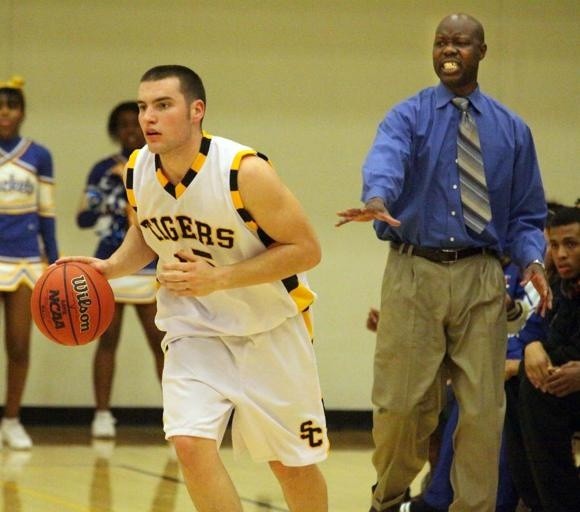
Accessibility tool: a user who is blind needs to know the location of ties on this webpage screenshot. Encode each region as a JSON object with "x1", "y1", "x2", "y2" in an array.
[
  {"x1": 390, "y1": 239, "x2": 496, "y2": 262},
  {"x1": 452, "y1": 97, "x2": 491, "y2": 234}
]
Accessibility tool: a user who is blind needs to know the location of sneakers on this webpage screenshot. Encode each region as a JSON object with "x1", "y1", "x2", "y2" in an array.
[
  {"x1": 1, "y1": 419, "x2": 31, "y2": 450},
  {"x1": 90, "y1": 407, "x2": 119, "y2": 439}
]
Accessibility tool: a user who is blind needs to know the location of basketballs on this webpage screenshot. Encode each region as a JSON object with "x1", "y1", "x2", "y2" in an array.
[{"x1": 31, "y1": 260, "x2": 115, "y2": 346}]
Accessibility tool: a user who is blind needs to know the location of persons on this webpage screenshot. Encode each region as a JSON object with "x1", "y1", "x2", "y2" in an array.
[
  {"x1": 54, "y1": 65, "x2": 331, "y2": 511},
  {"x1": 335, "y1": 12, "x2": 554, "y2": 511},
  {"x1": 75, "y1": 102, "x2": 166, "y2": 438},
  {"x1": 1, "y1": 87, "x2": 58, "y2": 451}
]
